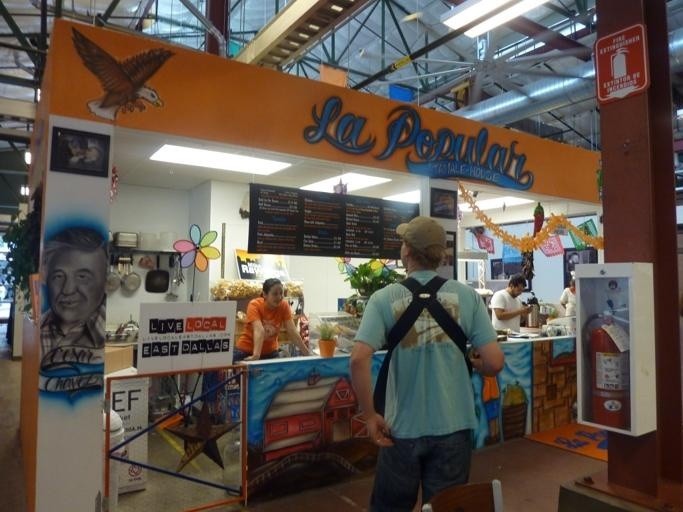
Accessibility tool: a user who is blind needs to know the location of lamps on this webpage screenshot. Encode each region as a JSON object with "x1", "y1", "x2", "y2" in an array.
[{"x1": 441, "y1": 0, "x2": 549, "y2": 38}]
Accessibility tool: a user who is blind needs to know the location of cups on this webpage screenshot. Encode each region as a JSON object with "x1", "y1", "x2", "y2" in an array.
[
  {"x1": 540, "y1": 306, "x2": 555, "y2": 315},
  {"x1": 547, "y1": 325, "x2": 557, "y2": 337}
]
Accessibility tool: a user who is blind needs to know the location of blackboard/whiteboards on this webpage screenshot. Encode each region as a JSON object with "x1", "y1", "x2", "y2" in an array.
[
  {"x1": 344, "y1": 194, "x2": 383, "y2": 260},
  {"x1": 247, "y1": 183, "x2": 304, "y2": 257},
  {"x1": 382, "y1": 199, "x2": 418, "y2": 260},
  {"x1": 302, "y1": 189, "x2": 344, "y2": 257}
]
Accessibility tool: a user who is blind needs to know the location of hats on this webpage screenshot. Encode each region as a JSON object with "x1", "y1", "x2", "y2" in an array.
[{"x1": 395, "y1": 215, "x2": 447, "y2": 250}]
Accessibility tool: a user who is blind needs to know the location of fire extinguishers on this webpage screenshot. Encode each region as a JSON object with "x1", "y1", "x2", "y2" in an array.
[{"x1": 582, "y1": 299, "x2": 630, "y2": 429}]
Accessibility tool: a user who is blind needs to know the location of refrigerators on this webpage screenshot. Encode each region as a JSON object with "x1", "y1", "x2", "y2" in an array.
[{"x1": 104, "y1": 367, "x2": 150, "y2": 495}]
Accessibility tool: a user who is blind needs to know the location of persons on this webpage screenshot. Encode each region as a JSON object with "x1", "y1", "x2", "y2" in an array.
[
  {"x1": 558, "y1": 279, "x2": 577, "y2": 317},
  {"x1": 489, "y1": 273, "x2": 534, "y2": 335},
  {"x1": 39, "y1": 227, "x2": 111, "y2": 400},
  {"x1": 84, "y1": 138, "x2": 106, "y2": 170},
  {"x1": 233, "y1": 278, "x2": 319, "y2": 361},
  {"x1": 349, "y1": 215, "x2": 505, "y2": 512}
]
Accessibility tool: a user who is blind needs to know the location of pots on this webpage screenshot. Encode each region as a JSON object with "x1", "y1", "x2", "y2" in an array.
[
  {"x1": 145, "y1": 255, "x2": 170, "y2": 293},
  {"x1": 108, "y1": 254, "x2": 141, "y2": 292}
]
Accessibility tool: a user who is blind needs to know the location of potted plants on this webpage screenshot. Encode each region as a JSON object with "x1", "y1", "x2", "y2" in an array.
[{"x1": 313, "y1": 321, "x2": 342, "y2": 357}]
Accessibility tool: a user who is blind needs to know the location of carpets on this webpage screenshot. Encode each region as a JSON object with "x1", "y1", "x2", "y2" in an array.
[{"x1": 523, "y1": 424, "x2": 609, "y2": 462}]
